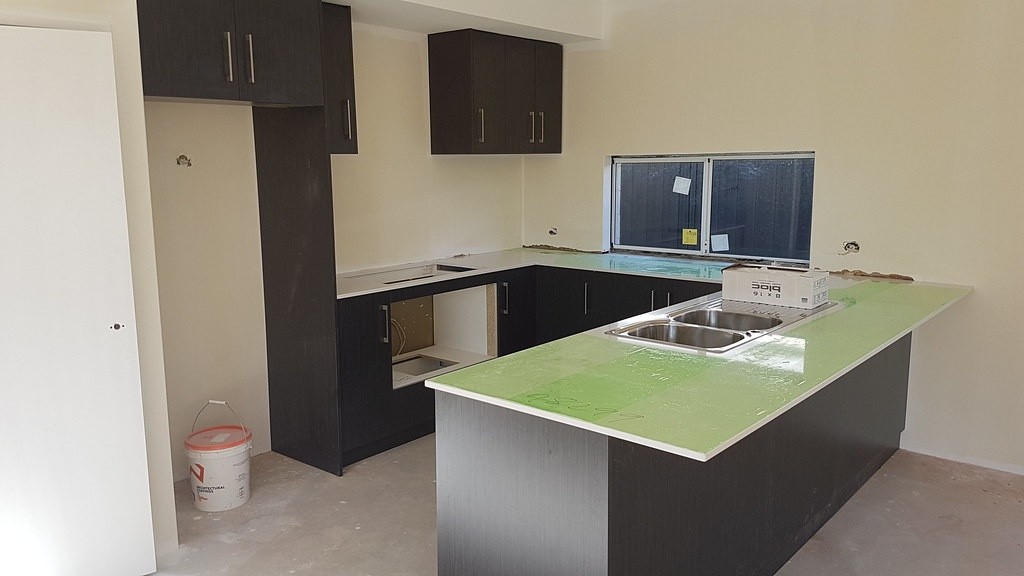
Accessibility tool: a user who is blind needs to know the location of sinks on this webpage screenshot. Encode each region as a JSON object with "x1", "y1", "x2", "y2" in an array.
[
  {"x1": 666, "y1": 309, "x2": 783, "y2": 334},
  {"x1": 604, "y1": 320, "x2": 744, "y2": 353}
]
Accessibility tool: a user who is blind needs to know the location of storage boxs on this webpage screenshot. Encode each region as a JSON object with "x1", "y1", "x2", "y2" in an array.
[{"x1": 722, "y1": 263, "x2": 829, "y2": 309}]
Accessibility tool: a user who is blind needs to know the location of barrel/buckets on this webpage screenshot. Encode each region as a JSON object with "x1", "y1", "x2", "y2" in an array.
[{"x1": 184, "y1": 399, "x2": 252, "y2": 512}]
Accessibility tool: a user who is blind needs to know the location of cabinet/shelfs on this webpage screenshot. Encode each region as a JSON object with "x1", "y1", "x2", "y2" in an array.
[
  {"x1": 136, "y1": 0, "x2": 325, "y2": 107},
  {"x1": 337, "y1": 245, "x2": 721, "y2": 478},
  {"x1": 427, "y1": 28, "x2": 562, "y2": 156},
  {"x1": 322, "y1": 1, "x2": 359, "y2": 155}
]
